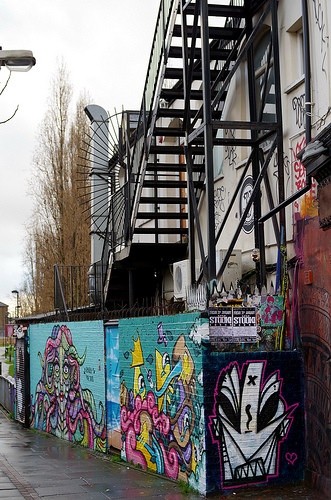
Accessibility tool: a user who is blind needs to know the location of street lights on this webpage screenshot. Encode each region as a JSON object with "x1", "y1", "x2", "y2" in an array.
[
  {"x1": 15, "y1": 306, "x2": 21, "y2": 318},
  {"x1": 12, "y1": 290, "x2": 19, "y2": 317}
]
